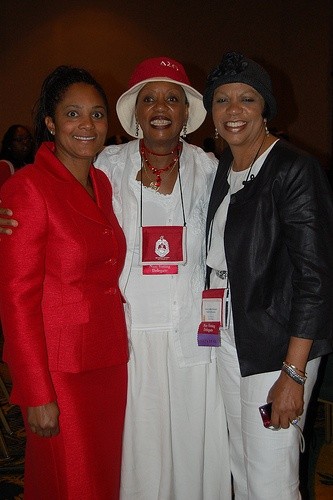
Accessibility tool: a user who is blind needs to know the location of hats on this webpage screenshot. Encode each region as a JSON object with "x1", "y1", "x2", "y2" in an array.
[
  {"x1": 203, "y1": 51, "x2": 277, "y2": 119},
  {"x1": 116, "y1": 56, "x2": 207, "y2": 138}
]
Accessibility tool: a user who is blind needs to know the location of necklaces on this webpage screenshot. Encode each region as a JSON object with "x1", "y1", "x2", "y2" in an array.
[{"x1": 140, "y1": 137, "x2": 181, "y2": 191}]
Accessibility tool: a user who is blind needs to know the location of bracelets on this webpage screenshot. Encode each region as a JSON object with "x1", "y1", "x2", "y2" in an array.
[{"x1": 282, "y1": 361, "x2": 308, "y2": 386}]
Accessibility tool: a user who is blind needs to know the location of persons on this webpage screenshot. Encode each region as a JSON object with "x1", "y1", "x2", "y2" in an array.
[
  {"x1": 200, "y1": 57, "x2": 333, "y2": 500},
  {"x1": 0, "y1": 65, "x2": 132, "y2": 500},
  {"x1": 0, "y1": 58, "x2": 234, "y2": 500}
]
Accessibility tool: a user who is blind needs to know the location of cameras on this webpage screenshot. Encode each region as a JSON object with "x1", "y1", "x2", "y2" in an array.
[{"x1": 260, "y1": 403, "x2": 273, "y2": 428}]
